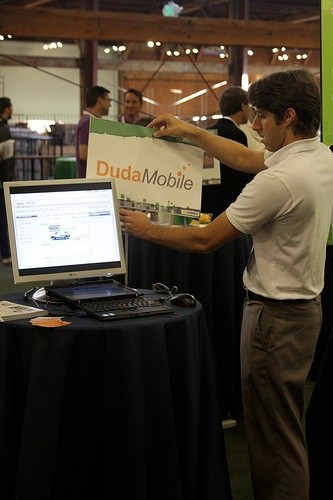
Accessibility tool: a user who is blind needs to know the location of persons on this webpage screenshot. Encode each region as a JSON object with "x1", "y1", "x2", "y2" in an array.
[
  {"x1": 119, "y1": 88, "x2": 143, "y2": 124},
  {"x1": 119, "y1": 68, "x2": 333, "y2": 500},
  {"x1": 205, "y1": 86, "x2": 248, "y2": 147},
  {"x1": 75, "y1": 86, "x2": 112, "y2": 178},
  {"x1": 0, "y1": 98, "x2": 15, "y2": 182},
  {"x1": 239, "y1": 105, "x2": 265, "y2": 149}
]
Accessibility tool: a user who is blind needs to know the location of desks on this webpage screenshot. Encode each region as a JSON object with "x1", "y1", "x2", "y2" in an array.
[
  {"x1": 11, "y1": 131, "x2": 55, "y2": 180},
  {"x1": 0, "y1": 285, "x2": 233, "y2": 500},
  {"x1": 52, "y1": 156, "x2": 79, "y2": 180},
  {"x1": 77, "y1": 220, "x2": 256, "y2": 436}
]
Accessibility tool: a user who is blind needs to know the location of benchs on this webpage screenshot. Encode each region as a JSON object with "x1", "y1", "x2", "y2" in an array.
[{"x1": 14, "y1": 146, "x2": 54, "y2": 182}]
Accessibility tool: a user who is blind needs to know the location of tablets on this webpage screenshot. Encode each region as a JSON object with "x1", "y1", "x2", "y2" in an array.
[{"x1": 42, "y1": 279, "x2": 144, "y2": 304}]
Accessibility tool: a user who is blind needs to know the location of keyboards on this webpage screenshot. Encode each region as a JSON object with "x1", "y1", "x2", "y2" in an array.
[{"x1": 76, "y1": 297, "x2": 175, "y2": 321}]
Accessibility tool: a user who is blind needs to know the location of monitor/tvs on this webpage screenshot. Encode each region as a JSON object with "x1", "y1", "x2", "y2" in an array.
[{"x1": 3, "y1": 176, "x2": 127, "y2": 304}]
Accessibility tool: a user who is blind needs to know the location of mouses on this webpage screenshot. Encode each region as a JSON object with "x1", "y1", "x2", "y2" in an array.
[{"x1": 172, "y1": 292, "x2": 196, "y2": 308}]
